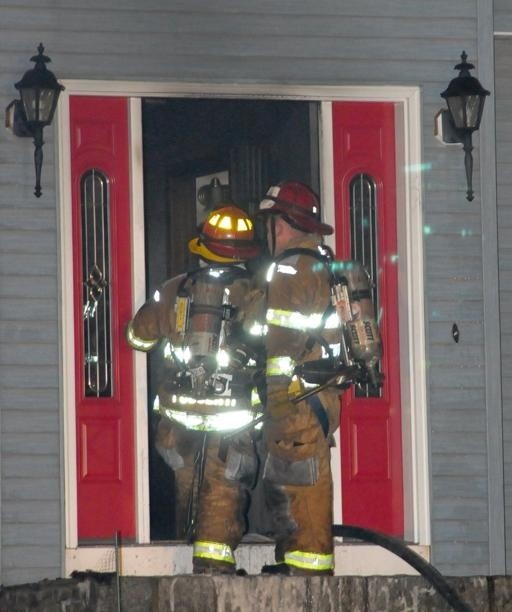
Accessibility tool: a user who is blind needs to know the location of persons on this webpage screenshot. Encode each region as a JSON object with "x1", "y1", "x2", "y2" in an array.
[
  {"x1": 257, "y1": 180, "x2": 344, "y2": 576},
  {"x1": 125, "y1": 203, "x2": 265, "y2": 574}
]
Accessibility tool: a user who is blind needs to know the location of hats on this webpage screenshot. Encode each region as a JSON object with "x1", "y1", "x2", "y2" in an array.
[
  {"x1": 188, "y1": 204, "x2": 262, "y2": 264},
  {"x1": 259, "y1": 180, "x2": 335, "y2": 235}
]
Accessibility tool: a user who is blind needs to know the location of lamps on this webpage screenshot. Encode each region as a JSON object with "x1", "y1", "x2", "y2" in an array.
[
  {"x1": 3, "y1": 41, "x2": 66, "y2": 198},
  {"x1": 434, "y1": 50, "x2": 491, "y2": 200}
]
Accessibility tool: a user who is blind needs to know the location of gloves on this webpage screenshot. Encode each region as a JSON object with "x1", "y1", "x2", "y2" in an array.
[{"x1": 266, "y1": 390, "x2": 296, "y2": 419}]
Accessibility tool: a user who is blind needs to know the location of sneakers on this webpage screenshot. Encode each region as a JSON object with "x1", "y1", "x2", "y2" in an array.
[
  {"x1": 261, "y1": 563, "x2": 334, "y2": 576},
  {"x1": 236, "y1": 569, "x2": 247, "y2": 576}
]
